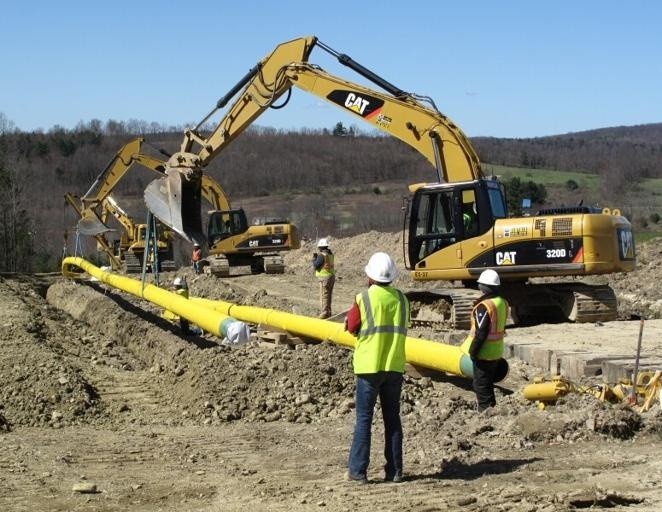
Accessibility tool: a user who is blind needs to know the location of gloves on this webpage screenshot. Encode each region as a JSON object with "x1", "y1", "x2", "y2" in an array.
[
  {"x1": 313, "y1": 253, "x2": 319, "y2": 258},
  {"x1": 469, "y1": 354, "x2": 480, "y2": 363}
]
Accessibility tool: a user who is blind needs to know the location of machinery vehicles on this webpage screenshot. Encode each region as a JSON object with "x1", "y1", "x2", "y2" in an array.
[
  {"x1": 93, "y1": 195, "x2": 175, "y2": 274},
  {"x1": 59, "y1": 134, "x2": 303, "y2": 284},
  {"x1": 140, "y1": 33, "x2": 641, "y2": 336}
]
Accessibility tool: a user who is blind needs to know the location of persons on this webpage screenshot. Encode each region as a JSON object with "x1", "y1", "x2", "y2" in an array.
[
  {"x1": 190, "y1": 243, "x2": 203, "y2": 276},
  {"x1": 460, "y1": 268, "x2": 510, "y2": 411},
  {"x1": 173, "y1": 277, "x2": 190, "y2": 333},
  {"x1": 432, "y1": 212, "x2": 471, "y2": 252},
  {"x1": 311, "y1": 238, "x2": 335, "y2": 319},
  {"x1": 342, "y1": 251, "x2": 412, "y2": 484}
]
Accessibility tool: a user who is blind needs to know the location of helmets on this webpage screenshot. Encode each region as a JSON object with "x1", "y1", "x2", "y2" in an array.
[
  {"x1": 477, "y1": 269, "x2": 501, "y2": 286},
  {"x1": 317, "y1": 238, "x2": 330, "y2": 248},
  {"x1": 365, "y1": 252, "x2": 398, "y2": 282},
  {"x1": 194, "y1": 242, "x2": 200, "y2": 247},
  {"x1": 174, "y1": 278, "x2": 183, "y2": 286}
]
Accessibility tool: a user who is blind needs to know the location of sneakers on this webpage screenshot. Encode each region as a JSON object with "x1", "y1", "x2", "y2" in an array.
[
  {"x1": 343, "y1": 471, "x2": 369, "y2": 486},
  {"x1": 379, "y1": 469, "x2": 403, "y2": 482}
]
